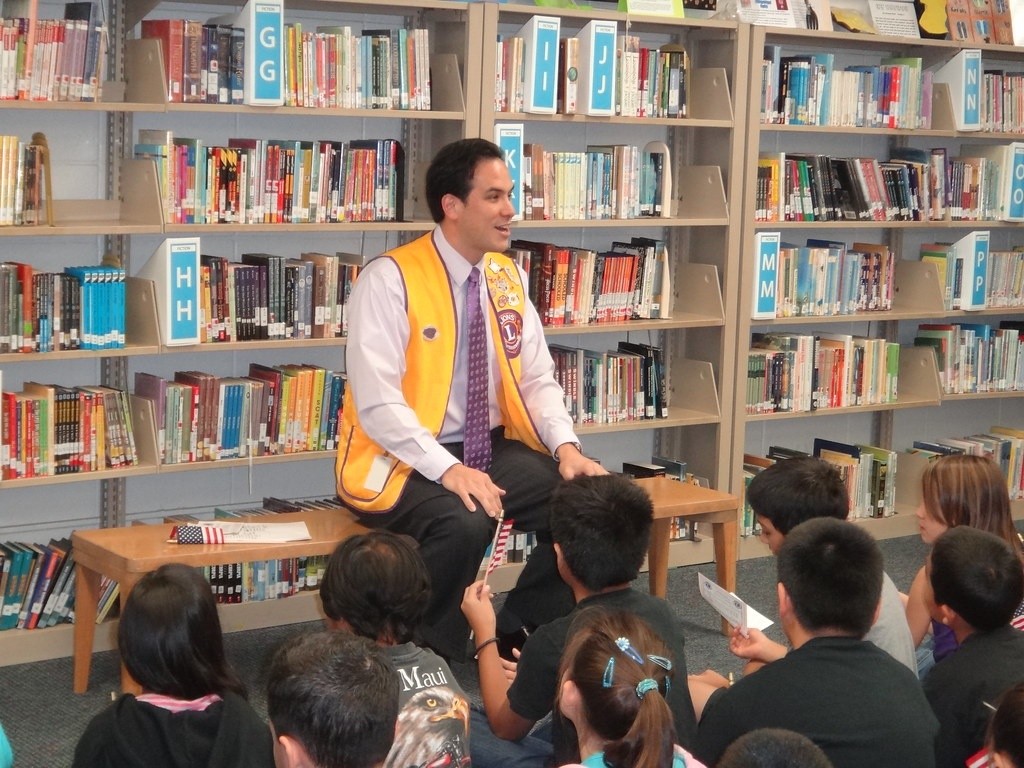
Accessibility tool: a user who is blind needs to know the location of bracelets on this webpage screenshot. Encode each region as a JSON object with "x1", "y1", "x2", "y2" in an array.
[{"x1": 472, "y1": 636, "x2": 501, "y2": 654}]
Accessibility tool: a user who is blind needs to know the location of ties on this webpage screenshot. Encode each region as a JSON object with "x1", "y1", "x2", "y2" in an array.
[{"x1": 463, "y1": 267, "x2": 491, "y2": 473}]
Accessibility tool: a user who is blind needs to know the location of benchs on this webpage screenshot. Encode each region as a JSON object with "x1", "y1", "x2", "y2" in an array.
[{"x1": 67, "y1": 474, "x2": 743, "y2": 699}]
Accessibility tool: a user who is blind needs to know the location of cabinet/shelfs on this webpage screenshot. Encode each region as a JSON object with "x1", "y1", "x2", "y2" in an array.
[{"x1": 2, "y1": 0, "x2": 1024, "y2": 674}]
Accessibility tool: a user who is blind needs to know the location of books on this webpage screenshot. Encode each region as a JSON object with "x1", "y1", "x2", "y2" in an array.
[
  {"x1": 0, "y1": 1, "x2": 709, "y2": 634},
  {"x1": 743, "y1": 0, "x2": 1024, "y2": 542}
]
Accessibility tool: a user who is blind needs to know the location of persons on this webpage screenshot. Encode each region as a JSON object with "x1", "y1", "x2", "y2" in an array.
[
  {"x1": 896, "y1": 451, "x2": 1024, "y2": 654},
  {"x1": 727, "y1": 455, "x2": 922, "y2": 682},
  {"x1": 909, "y1": 525, "x2": 1024, "y2": 768},
  {"x1": 703, "y1": 515, "x2": 942, "y2": 767},
  {"x1": 71, "y1": 562, "x2": 274, "y2": 768},
  {"x1": 458, "y1": 473, "x2": 700, "y2": 768},
  {"x1": 555, "y1": 605, "x2": 712, "y2": 768},
  {"x1": 316, "y1": 530, "x2": 475, "y2": 767},
  {"x1": 263, "y1": 624, "x2": 400, "y2": 768},
  {"x1": 346, "y1": 138, "x2": 612, "y2": 663},
  {"x1": 989, "y1": 678, "x2": 1024, "y2": 768}
]
